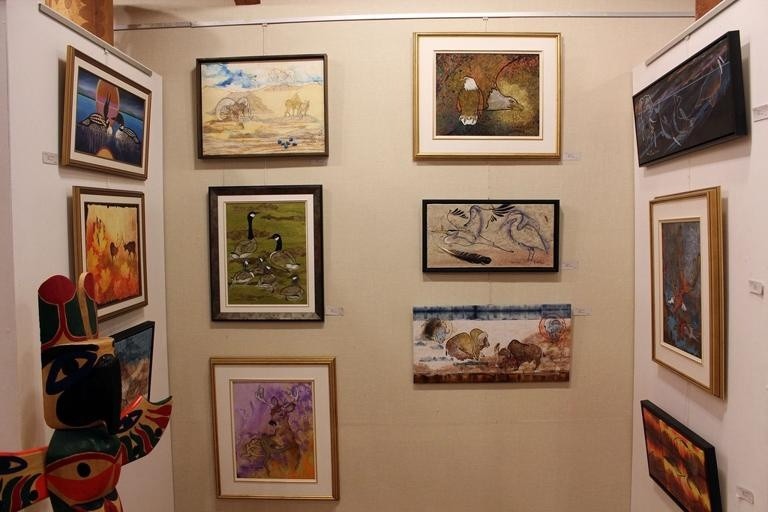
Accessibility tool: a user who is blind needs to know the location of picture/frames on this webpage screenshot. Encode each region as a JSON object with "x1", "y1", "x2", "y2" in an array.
[
  {"x1": 195, "y1": 53, "x2": 330, "y2": 162},
  {"x1": 648, "y1": 186, "x2": 730, "y2": 401},
  {"x1": 207, "y1": 184, "x2": 326, "y2": 324},
  {"x1": 421, "y1": 197, "x2": 561, "y2": 274},
  {"x1": 208, "y1": 354, "x2": 342, "y2": 502},
  {"x1": 629, "y1": 31, "x2": 749, "y2": 169},
  {"x1": 639, "y1": 399, "x2": 721, "y2": 512},
  {"x1": 61, "y1": 45, "x2": 155, "y2": 420},
  {"x1": 411, "y1": 29, "x2": 564, "y2": 163}
]
